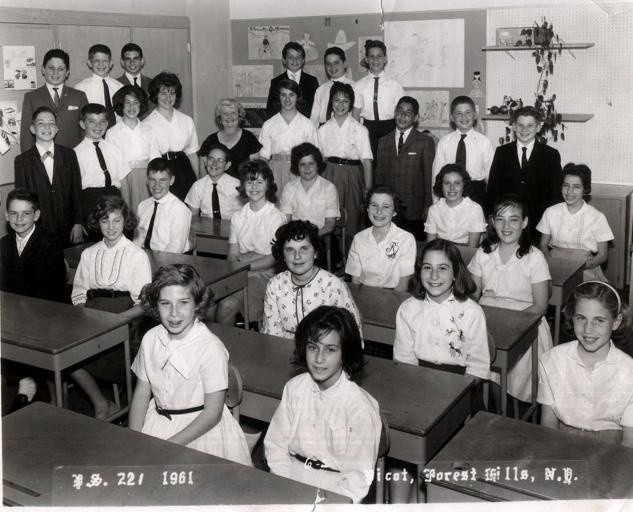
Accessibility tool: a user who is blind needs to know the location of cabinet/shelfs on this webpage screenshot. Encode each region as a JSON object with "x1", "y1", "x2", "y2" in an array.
[
  {"x1": 0, "y1": 11, "x2": 129, "y2": 196},
  {"x1": 128, "y1": 14, "x2": 191, "y2": 125},
  {"x1": 482, "y1": 43, "x2": 595, "y2": 123}
]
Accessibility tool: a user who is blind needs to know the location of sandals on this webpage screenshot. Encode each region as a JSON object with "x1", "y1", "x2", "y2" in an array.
[{"x1": 95, "y1": 401, "x2": 120, "y2": 421}]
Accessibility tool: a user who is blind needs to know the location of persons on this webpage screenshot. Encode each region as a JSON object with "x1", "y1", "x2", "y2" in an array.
[{"x1": 1, "y1": 40, "x2": 633, "y2": 505}]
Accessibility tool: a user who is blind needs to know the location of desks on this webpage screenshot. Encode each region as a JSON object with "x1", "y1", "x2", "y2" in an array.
[{"x1": 1, "y1": 183, "x2": 633, "y2": 507}]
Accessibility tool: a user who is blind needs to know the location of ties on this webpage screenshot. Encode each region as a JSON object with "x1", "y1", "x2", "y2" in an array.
[
  {"x1": 399, "y1": 133, "x2": 403, "y2": 153},
  {"x1": 212, "y1": 184, "x2": 221, "y2": 220},
  {"x1": 53, "y1": 88, "x2": 59, "y2": 107},
  {"x1": 93, "y1": 141, "x2": 111, "y2": 187},
  {"x1": 456, "y1": 134, "x2": 467, "y2": 171},
  {"x1": 133, "y1": 77, "x2": 138, "y2": 87},
  {"x1": 144, "y1": 201, "x2": 158, "y2": 250},
  {"x1": 373, "y1": 77, "x2": 378, "y2": 120},
  {"x1": 102, "y1": 79, "x2": 116, "y2": 128},
  {"x1": 521, "y1": 147, "x2": 527, "y2": 167}
]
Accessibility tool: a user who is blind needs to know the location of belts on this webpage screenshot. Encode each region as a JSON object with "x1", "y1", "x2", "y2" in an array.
[
  {"x1": 154, "y1": 401, "x2": 204, "y2": 421},
  {"x1": 327, "y1": 156, "x2": 362, "y2": 166},
  {"x1": 290, "y1": 452, "x2": 340, "y2": 473},
  {"x1": 87, "y1": 288, "x2": 129, "y2": 298},
  {"x1": 163, "y1": 151, "x2": 185, "y2": 161}
]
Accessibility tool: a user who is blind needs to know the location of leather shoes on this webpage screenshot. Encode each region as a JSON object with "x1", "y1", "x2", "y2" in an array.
[{"x1": 7, "y1": 382, "x2": 42, "y2": 415}]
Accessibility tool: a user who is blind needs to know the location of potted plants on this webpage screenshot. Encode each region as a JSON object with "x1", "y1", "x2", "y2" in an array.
[{"x1": 494, "y1": 20, "x2": 576, "y2": 148}]
